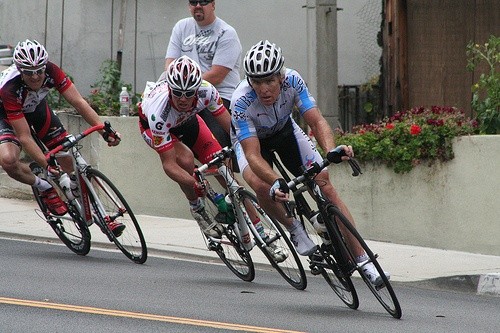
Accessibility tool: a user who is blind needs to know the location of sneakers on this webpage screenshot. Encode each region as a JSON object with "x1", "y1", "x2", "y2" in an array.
[
  {"x1": 291, "y1": 224, "x2": 317, "y2": 256},
  {"x1": 357, "y1": 260, "x2": 390, "y2": 287},
  {"x1": 37, "y1": 178, "x2": 68, "y2": 216},
  {"x1": 190, "y1": 204, "x2": 221, "y2": 238},
  {"x1": 269, "y1": 244, "x2": 289, "y2": 262},
  {"x1": 104, "y1": 216, "x2": 125, "y2": 235}
]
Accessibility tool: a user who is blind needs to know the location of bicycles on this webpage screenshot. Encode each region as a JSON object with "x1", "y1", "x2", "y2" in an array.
[
  {"x1": 270, "y1": 145, "x2": 402, "y2": 320},
  {"x1": 190, "y1": 150, "x2": 307, "y2": 290},
  {"x1": 28, "y1": 119, "x2": 148, "y2": 265}
]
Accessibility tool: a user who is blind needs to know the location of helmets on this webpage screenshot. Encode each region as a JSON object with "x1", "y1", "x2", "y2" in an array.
[
  {"x1": 242, "y1": 40, "x2": 285, "y2": 79},
  {"x1": 12, "y1": 39, "x2": 48, "y2": 67},
  {"x1": 166, "y1": 55, "x2": 201, "y2": 90}
]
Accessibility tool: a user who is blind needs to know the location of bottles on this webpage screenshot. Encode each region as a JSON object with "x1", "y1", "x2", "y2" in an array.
[
  {"x1": 58, "y1": 172, "x2": 76, "y2": 201},
  {"x1": 119, "y1": 86, "x2": 129, "y2": 118},
  {"x1": 69, "y1": 174, "x2": 80, "y2": 198},
  {"x1": 310, "y1": 209, "x2": 320, "y2": 225},
  {"x1": 211, "y1": 191, "x2": 236, "y2": 226},
  {"x1": 224, "y1": 193, "x2": 236, "y2": 226},
  {"x1": 313, "y1": 213, "x2": 332, "y2": 246}
]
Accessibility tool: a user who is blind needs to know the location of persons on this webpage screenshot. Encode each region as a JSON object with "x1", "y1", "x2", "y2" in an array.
[
  {"x1": 0, "y1": 39, "x2": 126, "y2": 234},
  {"x1": 166, "y1": 0, "x2": 243, "y2": 173},
  {"x1": 139, "y1": 55, "x2": 288, "y2": 260},
  {"x1": 230, "y1": 40, "x2": 390, "y2": 284}
]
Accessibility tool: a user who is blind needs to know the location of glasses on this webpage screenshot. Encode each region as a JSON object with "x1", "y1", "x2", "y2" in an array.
[
  {"x1": 19, "y1": 64, "x2": 46, "y2": 75},
  {"x1": 188, "y1": 0, "x2": 214, "y2": 6},
  {"x1": 171, "y1": 89, "x2": 196, "y2": 98}
]
